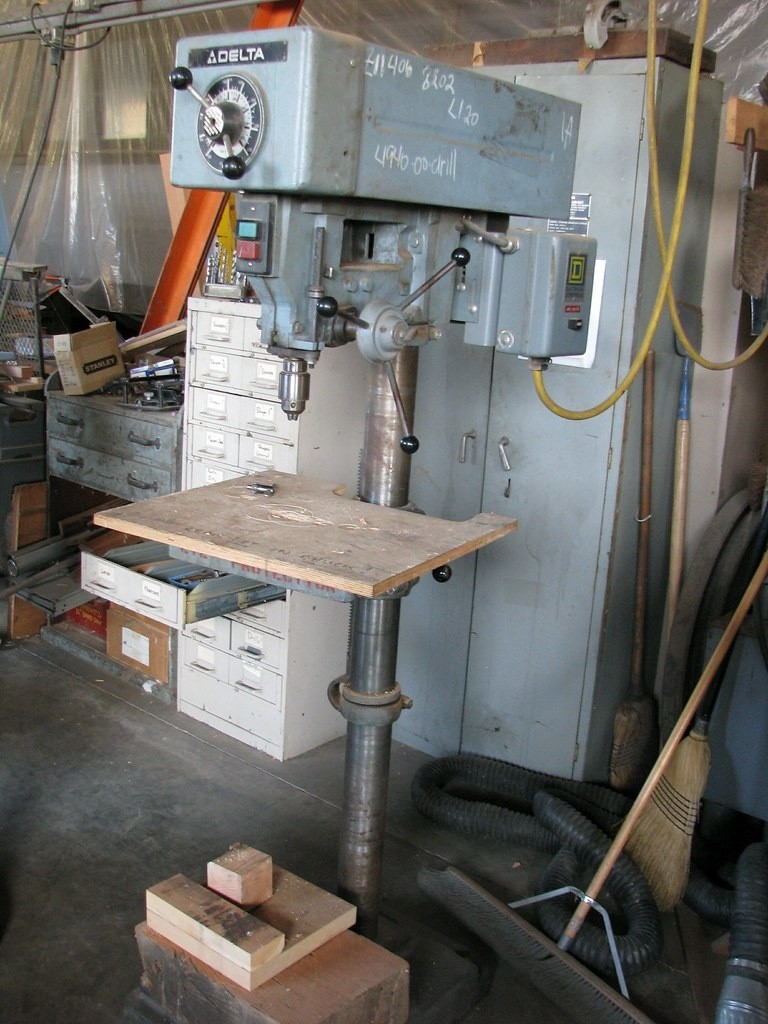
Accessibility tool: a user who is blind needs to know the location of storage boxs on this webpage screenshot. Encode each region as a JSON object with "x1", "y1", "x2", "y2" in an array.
[
  {"x1": 105, "y1": 609, "x2": 170, "y2": 685},
  {"x1": 124, "y1": 353, "x2": 175, "y2": 394},
  {"x1": 53, "y1": 321, "x2": 126, "y2": 395}
]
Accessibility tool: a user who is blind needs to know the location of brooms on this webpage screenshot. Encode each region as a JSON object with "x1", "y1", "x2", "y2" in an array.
[{"x1": 610, "y1": 349, "x2": 767, "y2": 910}]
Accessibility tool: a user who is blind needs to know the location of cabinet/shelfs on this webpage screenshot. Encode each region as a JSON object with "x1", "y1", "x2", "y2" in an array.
[{"x1": 178, "y1": 57, "x2": 726, "y2": 779}]
